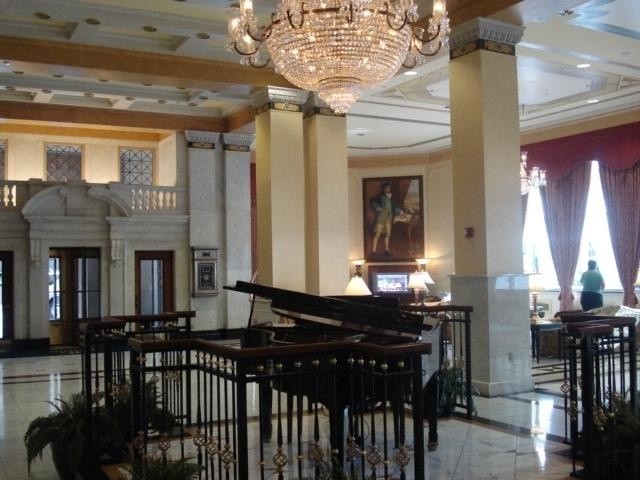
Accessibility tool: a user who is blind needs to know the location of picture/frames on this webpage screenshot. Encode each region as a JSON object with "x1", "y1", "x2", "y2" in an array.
[{"x1": 362, "y1": 175, "x2": 424, "y2": 262}]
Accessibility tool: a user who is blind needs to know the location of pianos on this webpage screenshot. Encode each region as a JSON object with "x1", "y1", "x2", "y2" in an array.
[{"x1": 223, "y1": 279, "x2": 432, "y2": 461}]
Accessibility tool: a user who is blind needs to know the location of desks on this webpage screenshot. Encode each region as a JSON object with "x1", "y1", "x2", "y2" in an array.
[{"x1": 531, "y1": 323, "x2": 562, "y2": 364}]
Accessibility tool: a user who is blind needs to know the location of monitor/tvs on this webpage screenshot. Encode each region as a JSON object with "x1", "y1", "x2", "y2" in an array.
[{"x1": 371, "y1": 270, "x2": 408, "y2": 296}]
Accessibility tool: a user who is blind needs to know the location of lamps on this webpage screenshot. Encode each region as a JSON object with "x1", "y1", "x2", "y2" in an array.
[
  {"x1": 408, "y1": 259, "x2": 435, "y2": 306},
  {"x1": 520, "y1": 150, "x2": 547, "y2": 197},
  {"x1": 224, "y1": 0, "x2": 451, "y2": 114},
  {"x1": 344, "y1": 259, "x2": 372, "y2": 295},
  {"x1": 529, "y1": 272, "x2": 545, "y2": 311}
]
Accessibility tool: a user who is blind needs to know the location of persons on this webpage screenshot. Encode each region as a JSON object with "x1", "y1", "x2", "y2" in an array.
[
  {"x1": 369, "y1": 180, "x2": 409, "y2": 256},
  {"x1": 580, "y1": 259, "x2": 605, "y2": 311}
]
